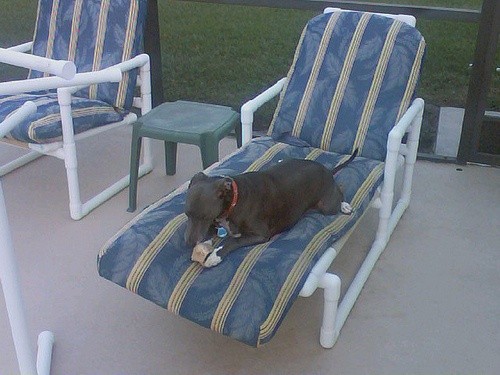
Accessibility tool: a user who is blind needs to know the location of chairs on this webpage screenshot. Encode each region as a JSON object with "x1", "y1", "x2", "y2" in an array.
[{"x1": 0, "y1": 1, "x2": 155, "y2": 221}]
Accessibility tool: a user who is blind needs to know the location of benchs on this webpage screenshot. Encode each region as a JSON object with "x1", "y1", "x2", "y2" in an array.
[{"x1": 95, "y1": 7, "x2": 426, "y2": 350}]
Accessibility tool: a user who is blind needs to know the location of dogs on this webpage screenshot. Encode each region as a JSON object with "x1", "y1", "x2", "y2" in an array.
[{"x1": 184, "y1": 147, "x2": 359, "y2": 268}]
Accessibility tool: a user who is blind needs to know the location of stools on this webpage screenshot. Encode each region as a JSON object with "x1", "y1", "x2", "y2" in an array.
[{"x1": 127, "y1": 99, "x2": 244, "y2": 212}]
